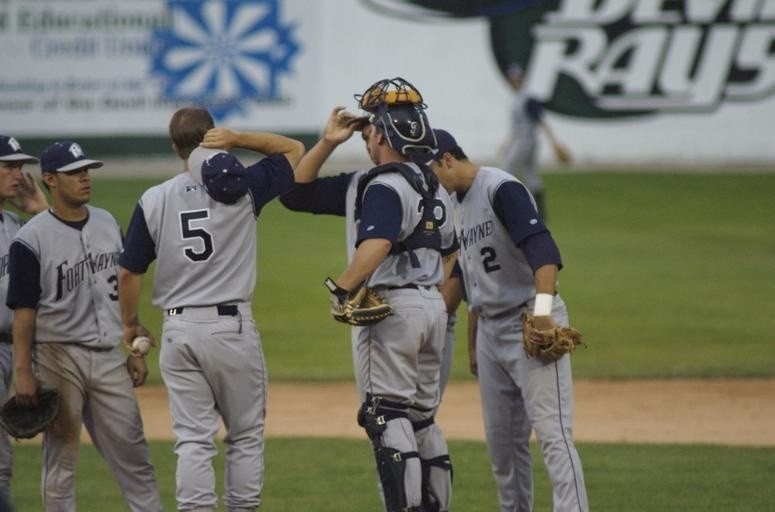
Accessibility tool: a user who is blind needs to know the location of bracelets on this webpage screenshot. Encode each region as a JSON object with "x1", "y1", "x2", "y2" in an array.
[{"x1": 533, "y1": 293, "x2": 553, "y2": 316}]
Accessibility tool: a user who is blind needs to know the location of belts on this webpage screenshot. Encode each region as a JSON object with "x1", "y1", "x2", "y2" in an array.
[
  {"x1": 168, "y1": 303, "x2": 238, "y2": 316},
  {"x1": 388, "y1": 282, "x2": 418, "y2": 290}
]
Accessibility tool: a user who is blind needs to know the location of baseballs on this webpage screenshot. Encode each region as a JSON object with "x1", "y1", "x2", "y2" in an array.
[{"x1": 132, "y1": 335, "x2": 151, "y2": 354}]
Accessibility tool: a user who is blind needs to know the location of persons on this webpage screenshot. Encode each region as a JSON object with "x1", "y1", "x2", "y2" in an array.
[
  {"x1": 0, "y1": 134, "x2": 53, "y2": 512},
  {"x1": 278, "y1": 76, "x2": 454, "y2": 512},
  {"x1": 5, "y1": 141, "x2": 166, "y2": 512},
  {"x1": 499, "y1": 66, "x2": 569, "y2": 212},
  {"x1": 424, "y1": 128, "x2": 587, "y2": 512},
  {"x1": 117, "y1": 106, "x2": 305, "y2": 512}
]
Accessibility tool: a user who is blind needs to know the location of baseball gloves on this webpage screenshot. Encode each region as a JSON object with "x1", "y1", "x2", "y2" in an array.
[
  {"x1": 325, "y1": 278, "x2": 393, "y2": 324},
  {"x1": 0, "y1": 389, "x2": 61, "y2": 441},
  {"x1": 522, "y1": 316, "x2": 587, "y2": 363}
]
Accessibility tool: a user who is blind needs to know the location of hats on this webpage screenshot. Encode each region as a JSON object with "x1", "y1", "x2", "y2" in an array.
[
  {"x1": 347, "y1": 104, "x2": 458, "y2": 157},
  {"x1": 40, "y1": 140, "x2": 103, "y2": 173},
  {"x1": 0, "y1": 134, "x2": 40, "y2": 164},
  {"x1": 187, "y1": 145, "x2": 248, "y2": 204}
]
